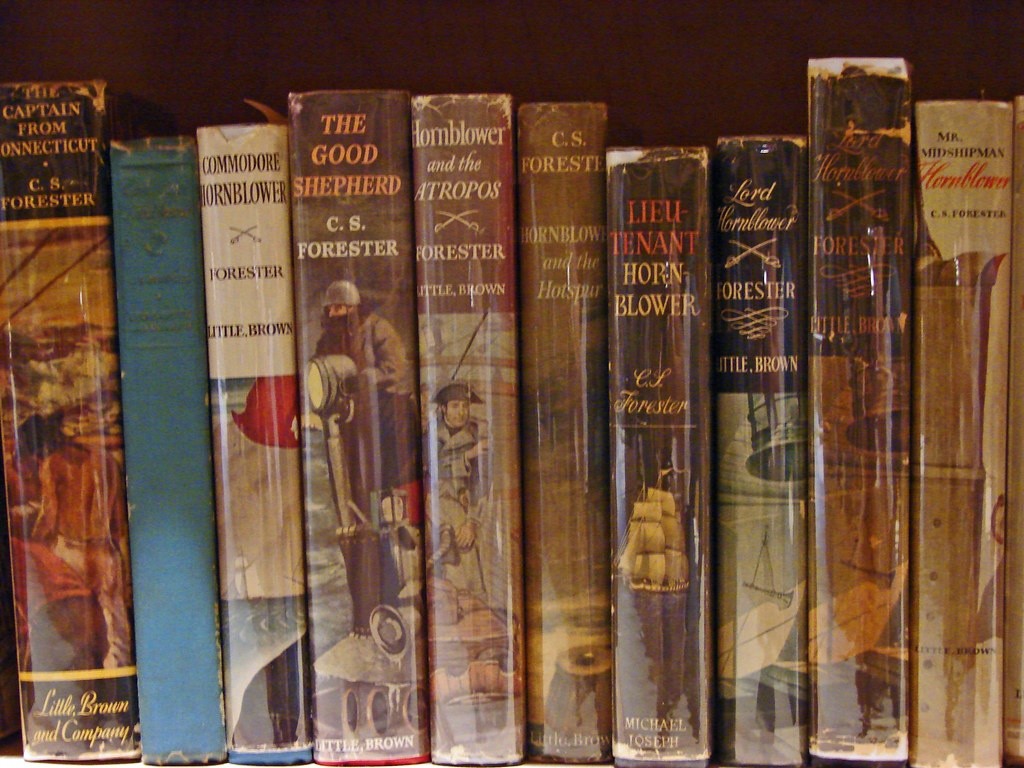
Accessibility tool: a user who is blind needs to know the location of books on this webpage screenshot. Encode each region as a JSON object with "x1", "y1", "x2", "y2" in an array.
[
  {"x1": 606, "y1": 146, "x2": 712, "y2": 768},
  {"x1": 287, "y1": 88, "x2": 432, "y2": 766},
  {"x1": 412, "y1": 92, "x2": 526, "y2": 764},
  {"x1": 106, "y1": 134, "x2": 228, "y2": 764},
  {"x1": 0, "y1": 80, "x2": 142, "y2": 761},
  {"x1": 713, "y1": 135, "x2": 808, "y2": 766},
  {"x1": 196, "y1": 124, "x2": 313, "y2": 764},
  {"x1": 808, "y1": 57, "x2": 914, "y2": 761},
  {"x1": 516, "y1": 102, "x2": 612, "y2": 763},
  {"x1": 910, "y1": 99, "x2": 1014, "y2": 768}
]
